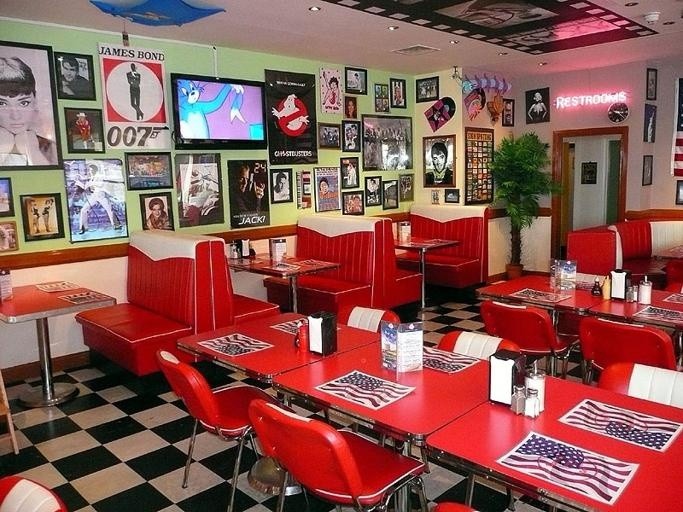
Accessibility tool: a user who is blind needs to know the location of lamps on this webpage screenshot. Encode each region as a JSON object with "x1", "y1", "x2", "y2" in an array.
[{"x1": 90, "y1": 0, "x2": 226, "y2": 46}]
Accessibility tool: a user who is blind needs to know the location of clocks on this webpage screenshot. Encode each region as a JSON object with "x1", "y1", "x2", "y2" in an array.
[{"x1": 608, "y1": 101, "x2": 630, "y2": 124}]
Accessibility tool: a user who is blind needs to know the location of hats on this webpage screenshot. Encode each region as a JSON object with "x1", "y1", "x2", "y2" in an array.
[{"x1": 76, "y1": 112, "x2": 88, "y2": 118}]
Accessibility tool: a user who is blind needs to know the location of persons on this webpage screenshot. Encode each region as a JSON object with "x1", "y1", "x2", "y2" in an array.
[
  {"x1": 72, "y1": 165, "x2": 122, "y2": 234},
  {"x1": 394, "y1": 86, "x2": 404, "y2": 105},
  {"x1": 427, "y1": 142, "x2": 453, "y2": 185},
  {"x1": 350, "y1": 196, "x2": 362, "y2": 213},
  {"x1": 274, "y1": 172, "x2": 291, "y2": 202},
  {"x1": 146, "y1": 198, "x2": 169, "y2": 229},
  {"x1": 59, "y1": 55, "x2": 90, "y2": 97},
  {"x1": 74, "y1": 112, "x2": 95, "y2": 149},
  {"x1": 347, "y1": 161, "x2": 357, "y2": 186},
  {"x1": 345, "y1": 125, "x2": 359, "y2": 150},
  {"x1": 365, "y1": 127, "x2": 409, "y2": 145},
  {"x1": 232, "y1": 164, "x2": 265, "y2": 226},
  {"x1": 0, "y1": 55, "x2": 57, "y2": 167},
  {"x1": 346, "y1": 98, "x2": 356, "y2": 118},
  {"x1": 29, "y1": 199, "x2": 54, "y2": 233},
  {"x1": 527, "y1": 92, "x2": 548, "y2": 120},
  {"x1": 323, "y1": 127, "x2": 339, "y2": 146},
  {"x1": 349, "y1": 73, "x2": 361, "y2": 90}
]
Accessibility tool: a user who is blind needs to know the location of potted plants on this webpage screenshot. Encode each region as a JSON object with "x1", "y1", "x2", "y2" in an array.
[{"x1": 486, "y1": 129, "x2": 565, "y2": 280}]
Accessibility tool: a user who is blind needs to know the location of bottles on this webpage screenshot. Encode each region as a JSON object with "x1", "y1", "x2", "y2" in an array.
[
  {"x1": 510, "y1": 370, "x2": 547, "y2": 418},
  {"x1": 396, "y1": 221, "x2": 412, "y2": 243},
  {"x1": 590, "y1": 275, "x2": 611, "y2": 300},
  {"x1": 270, "y1": 238, "x2": 287, "y2": 264},
  {"x1": 625, "y1": 276, "x2": 655, "y2": 305},
  {"x1": 230, "y1": 240, "x2": 241, "y2": 259}
]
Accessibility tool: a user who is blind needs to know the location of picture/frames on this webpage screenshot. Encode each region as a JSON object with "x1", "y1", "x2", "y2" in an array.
[
  {"x1": 643, "y1": 103, "x2": 657, "y2": 142},
  {"x1": 581, "y1": 162, "x2": 597, "y2": 184},
  {"x1": 642, "y1": 155, "x2": 653, "y2": 186},
  {"x1": 675, "y1": 180, "x2": 683, "y2": 205},
  {"x1": 646, "y1": 67, "x2": 658, "y2": 100}
]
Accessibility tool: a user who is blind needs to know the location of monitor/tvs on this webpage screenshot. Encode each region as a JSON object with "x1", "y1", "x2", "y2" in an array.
[{"x1": 171, "y1": 73, "x2": 267, "y2": 150}]
